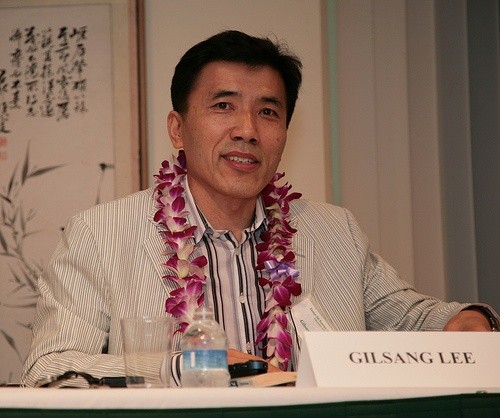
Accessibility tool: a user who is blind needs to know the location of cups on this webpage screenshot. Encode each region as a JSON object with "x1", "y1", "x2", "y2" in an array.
[{"x1": 121, "y1": 315, "x2": 173, "y2": 389}]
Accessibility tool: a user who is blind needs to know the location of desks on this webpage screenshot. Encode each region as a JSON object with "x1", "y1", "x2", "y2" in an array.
[{"x1": 0, "y1": 387, "x2": 500, "y2": 418}]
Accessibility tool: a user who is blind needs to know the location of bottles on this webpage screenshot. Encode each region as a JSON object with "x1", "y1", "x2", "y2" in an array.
[{"x1": 180, "y1": 306, "x2": 231, "y2": 389}]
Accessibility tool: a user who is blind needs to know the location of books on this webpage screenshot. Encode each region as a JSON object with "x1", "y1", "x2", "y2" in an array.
[{"x1": 230, "y1": 372, "x2": 297, "y2": 388}]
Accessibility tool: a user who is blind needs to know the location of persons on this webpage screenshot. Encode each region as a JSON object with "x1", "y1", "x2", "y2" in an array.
[{"x1": 21, "y1": 30, "x2": 500, "y2": 389}]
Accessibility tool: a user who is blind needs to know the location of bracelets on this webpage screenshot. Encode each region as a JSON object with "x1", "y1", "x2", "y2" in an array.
[{"x1": 461, "y1": 305, "x2": 497, "y2": 332}]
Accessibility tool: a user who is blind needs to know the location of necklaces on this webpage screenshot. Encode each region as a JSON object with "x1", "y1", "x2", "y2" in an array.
[{"x1": 151, "y1": 149, "x2": 302, "y2": 374}]
